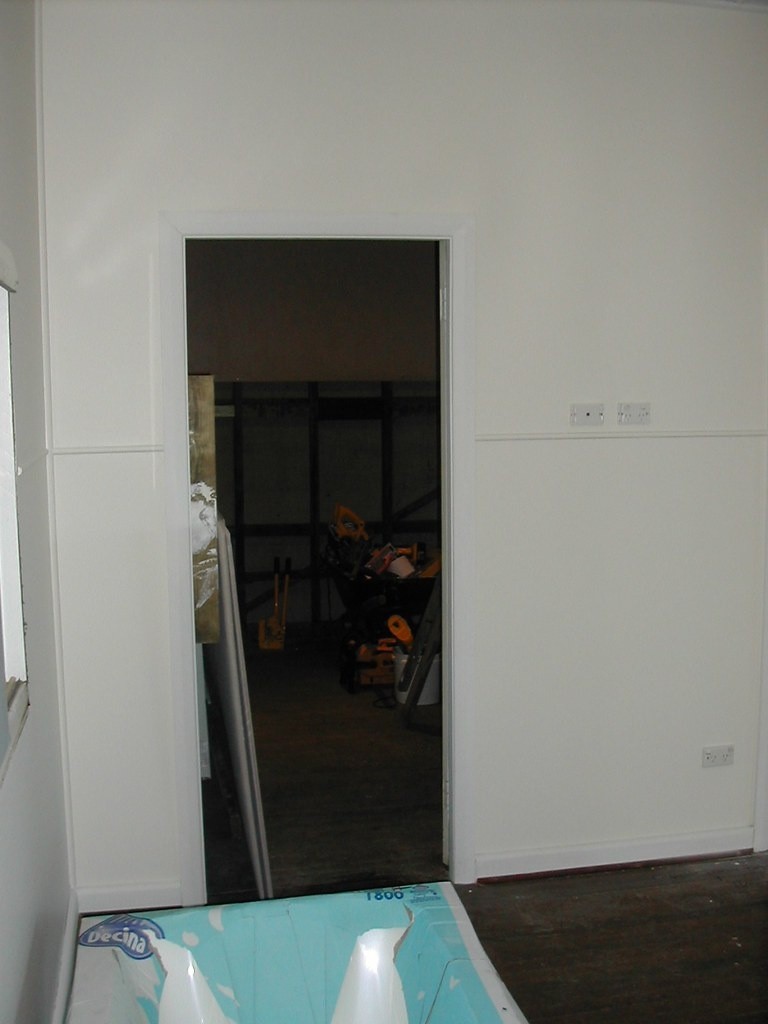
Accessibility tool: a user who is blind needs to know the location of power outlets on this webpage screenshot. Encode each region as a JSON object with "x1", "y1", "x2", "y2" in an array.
[{"x1": 617, "y1": 402, "x2": 651, "y2": 426}]
